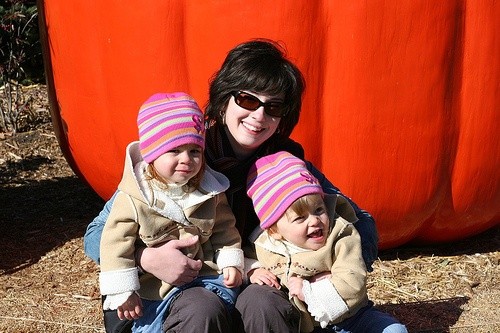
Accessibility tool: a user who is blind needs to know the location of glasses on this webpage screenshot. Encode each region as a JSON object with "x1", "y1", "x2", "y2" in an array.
[{"x1": 231, "y1": 90, "x2": 289, "y2": 116}]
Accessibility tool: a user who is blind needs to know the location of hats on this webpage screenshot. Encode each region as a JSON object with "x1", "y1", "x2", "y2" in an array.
[
  {"x1": 137, "y1": 91, "x2": 205, "y2": 163},
  {"x1": 247, "y1": 150, "x2": 324, "y2": 229}
]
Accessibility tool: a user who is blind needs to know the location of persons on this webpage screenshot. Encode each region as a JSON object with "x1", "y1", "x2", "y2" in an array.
[
  {"x1": 243, "y1": 151, "x2": 408, "y2": 333},
  {"x1": 99, "y1": 90, "x2": 245, "y2": 333},
  {"x1": 83, "y1": 37, "x2": 379, "y2": 333}
]
